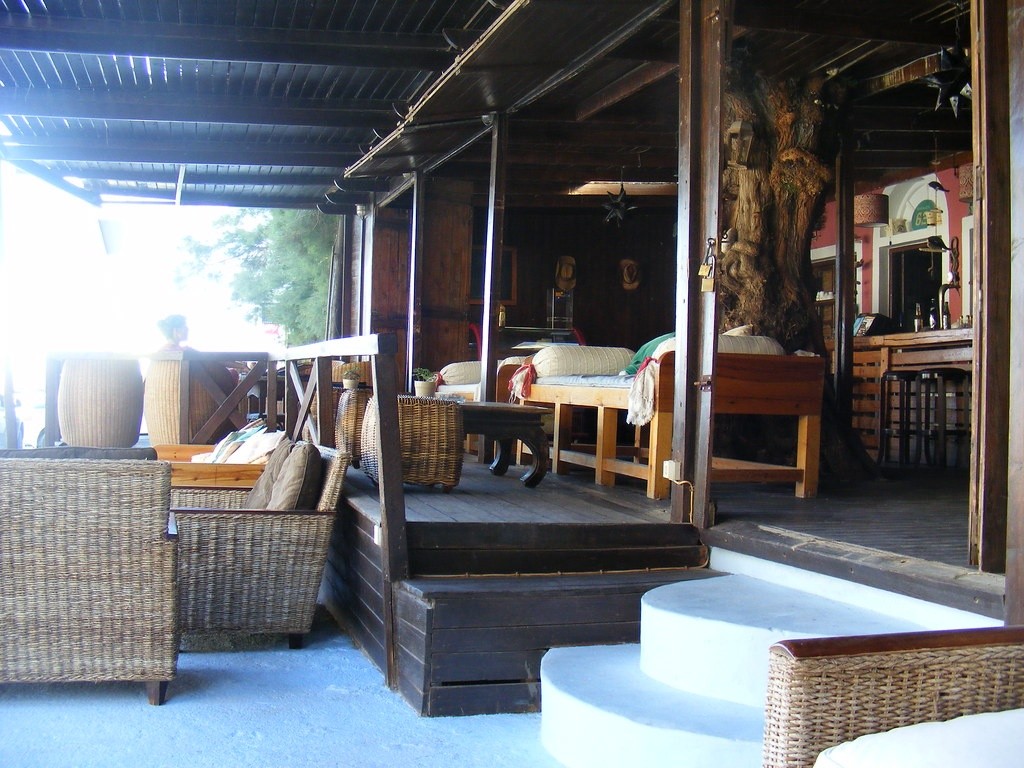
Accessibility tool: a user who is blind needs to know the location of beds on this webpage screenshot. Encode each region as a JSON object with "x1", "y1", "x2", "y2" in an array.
[
  {"x1": 432, "y1": 363, "x2": 589, "y2": 461},
  {"x1": 515, "y1": 351, "x2": 826, "y2": 500}
]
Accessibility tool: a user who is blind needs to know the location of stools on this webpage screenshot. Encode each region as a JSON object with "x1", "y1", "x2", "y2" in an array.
[
  {"x1": 913, "y1": 368, "x2": 970, "y2": 480},
  {"x1": 171, "y1": 439, "x2": 351, "y2": 650},
  {"x1": 876, "y1": 371, "x2": 931, "y2": 477},
  {"x1": 301, "y1": 388, "x2": 464, "y2": 494}
]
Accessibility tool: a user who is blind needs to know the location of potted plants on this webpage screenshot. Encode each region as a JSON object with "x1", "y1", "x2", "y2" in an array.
[
  {"x1": 340, "y1": 366, "x2": 363, "y2": 389},
  {"x1": 413, "y1": 367, "x2": 437, "y2": 397}
]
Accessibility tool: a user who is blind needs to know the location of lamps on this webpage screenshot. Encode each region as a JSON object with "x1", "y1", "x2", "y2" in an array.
[
  {"x1": 853, "y1": 176, "x2": 889, "y2": 227},
  {"x1": 959, "y1": 163, "x2": 973, "y2": 202}
]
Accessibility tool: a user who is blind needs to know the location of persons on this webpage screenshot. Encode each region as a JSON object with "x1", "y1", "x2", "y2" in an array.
[{"x1": 154, "y1": 315, "x2": 252, "y2": 385}]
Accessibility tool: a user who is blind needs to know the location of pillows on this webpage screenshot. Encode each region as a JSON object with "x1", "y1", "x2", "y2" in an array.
[
  {"x1": 434, "y1": 361, "x2": 508, "y2": 392},
  {"x1": 496, "y1": 356, "x2": 531, "y2": 375},
  {"x1": 508, "y1": 346, "x2": 635, "y2": 404},
  {"x1": 242, "y1": 436, "x2": 295, "y2": 508},
  {"x1": 266, "y1": 441, "x2": 322, "y2": 510},
  {"x1": 620, "y1": 324, "x2": 786, "y2": 426},
  {"x1": 191, "y1": 418, "x2": 287, "y2": 464}
]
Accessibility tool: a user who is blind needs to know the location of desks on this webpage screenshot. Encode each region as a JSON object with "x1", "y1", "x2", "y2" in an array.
[
  {"x1": 240, "y1": 374, "x2": 285, "y2": 431},
  {"x1": 423, "y1": 402, "x2": 554, "y2": 488}
]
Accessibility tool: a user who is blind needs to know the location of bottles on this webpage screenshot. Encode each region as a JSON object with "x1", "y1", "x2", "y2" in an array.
[
  {"x1": 942, "y1": 301, "x2": 952, "y2": 330},
  {"x1": 929, "y1": 299, "x2": 938, "y2": 330},
  {"x1": 913, "y1": 302, "x2": 924, "y2": 333}
]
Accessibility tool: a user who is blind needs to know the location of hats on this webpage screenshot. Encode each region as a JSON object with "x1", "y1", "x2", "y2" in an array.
[
  {"x1": 617, "y1": 259, "x2": 644, "y2": 291},
  {"x1": 555, "y1": 256, "x2": 577, "y2": 292}
]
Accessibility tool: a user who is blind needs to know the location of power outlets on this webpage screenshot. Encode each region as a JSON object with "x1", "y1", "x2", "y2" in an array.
[{"x1": 662, "y1": 460, "x2": 680, "y2": 480}]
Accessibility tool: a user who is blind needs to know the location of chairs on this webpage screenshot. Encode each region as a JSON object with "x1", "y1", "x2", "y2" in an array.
[
  {"x1": 154, "y1": 444, "x2": 271, "y2": 487},
  {"x1": 0, "y1": 458, "x2": 178, "y2": 706},
  {"x1": 263, "y1": 360, "x2": 344, "y2": 426},
  {"x1": 57, "y1": 355, "x2": 144, "y2": 448},
  {"x1": 296, "y1": 361, "x2": 400, "y2": 444},
  {"x1": 145, "y1": 360, "x2": 236, "y2": 447}
]
